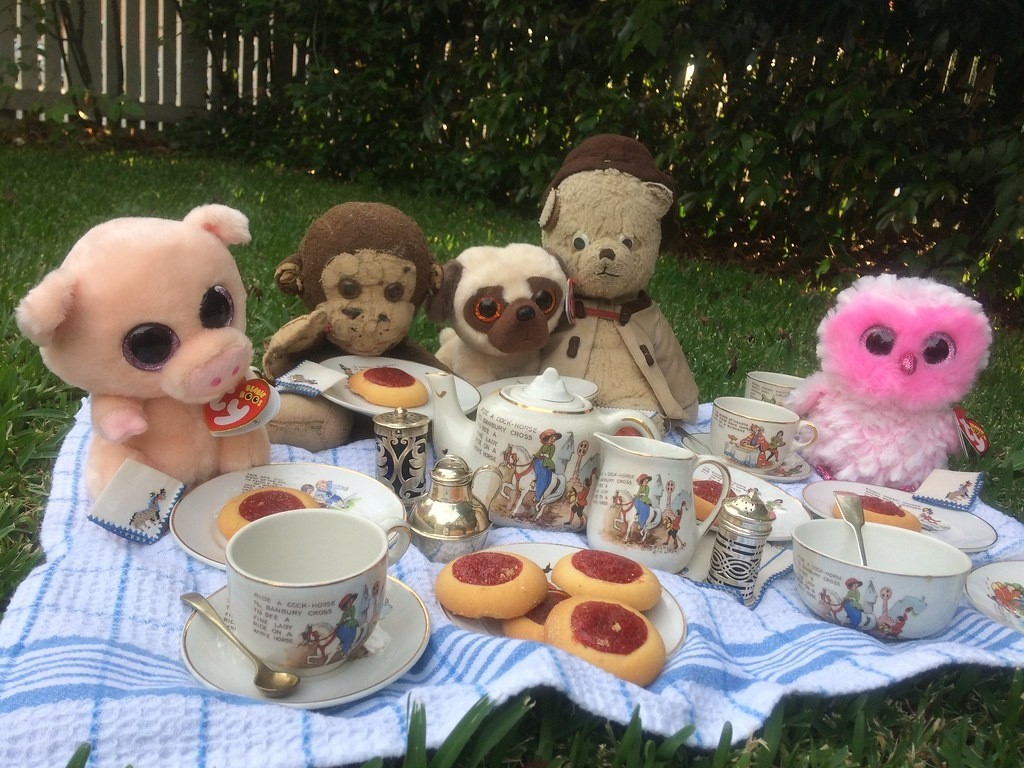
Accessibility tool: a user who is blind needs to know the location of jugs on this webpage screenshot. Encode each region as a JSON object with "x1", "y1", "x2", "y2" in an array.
[
  {"x1": 424, "y1": 366, "x2": 662, "y2": 533},
  {"x1": 406, "y1": 454, "x2": 504, "y2": 562}
]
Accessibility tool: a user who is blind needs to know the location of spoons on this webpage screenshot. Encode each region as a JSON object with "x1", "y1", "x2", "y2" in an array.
[{"x1": 180, "y1": 592, "x2": 301, "y2": 698}]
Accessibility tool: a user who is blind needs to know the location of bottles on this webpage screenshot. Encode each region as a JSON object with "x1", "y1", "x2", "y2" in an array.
[
  {"x1": 372, "y1": 406, "x2": 430, "y2": 509},
  {"x1": 703, "y1": 487, "x2": 772, "y2": 604}
]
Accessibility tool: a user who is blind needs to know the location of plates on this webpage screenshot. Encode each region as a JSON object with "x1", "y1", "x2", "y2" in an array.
[
  {"x1": 801, "y1": 479, "x2": 999, "y2": 554},
  {"x1": 315, "y1": 355, "x2": 482, "y2": 420},
  {"x1": 693, "y1": 457, "x2": 814, "y2": 543},
  {"x1": 181, "y1": 574, "x2": 431, "y2": 710},
  {"x1": 168, "y1": 462, "x2": 407, "y2": 570},
  {"x1": 680, "y1": 432, "x2": 812, "y2": 482},
  {"x1": 474, "y1": 374, "x2": 600, "y2": 400},
  {"x1": 961, "y1": 559, "x2": 1024, "y2": 637},
  {"x1": 437, "y1": 540, "x2": 686, "y2": 661}
]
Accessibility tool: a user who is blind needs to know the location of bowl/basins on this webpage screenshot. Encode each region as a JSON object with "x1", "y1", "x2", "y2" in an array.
[{"x1": 790, "y1": 518, "x2": 973, "y2": 641}]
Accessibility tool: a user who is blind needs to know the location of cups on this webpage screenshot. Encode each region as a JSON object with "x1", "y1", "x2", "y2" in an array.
[
  {"x1": 224, "y1": 508, "x2": 412, "y2": 678},
  {"x1": 710, "y1": 396, "x2": 819, "y2": 474},
  {"x1": 744, "y1": 371, "x2": 806, "y2": 406},
  {"x1": 587, "y1": 431, "x2": 730, "y2": 574}
]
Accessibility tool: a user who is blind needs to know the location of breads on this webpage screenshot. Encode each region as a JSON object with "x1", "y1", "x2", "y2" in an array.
[
  {"x1": 349, "y1": 365, "x2": 429, "y2": 408},
  {"x1": 832, "y1": 494, "x2": 921, "y2": 535},
  {"x1": 692, "y1": 478, "x2": 738, "y2": 527},
  {"x1": 218, "y1": 486, "x2": 322, "y2": 542},
  {"x1": 433, "y1": 550, "x2": 668, "y2": 689}
]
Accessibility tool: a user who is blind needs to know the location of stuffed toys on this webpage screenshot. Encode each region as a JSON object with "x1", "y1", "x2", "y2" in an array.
[
  {"x1": 782, "y1": 273, "x2": 993, "y2": 491},
  {"x1": 263, "y1": 134, "x2": 699, "y2": 453},
  {"x1": 14, "y1": 202, "x2": 271, "y2": 507}
]
